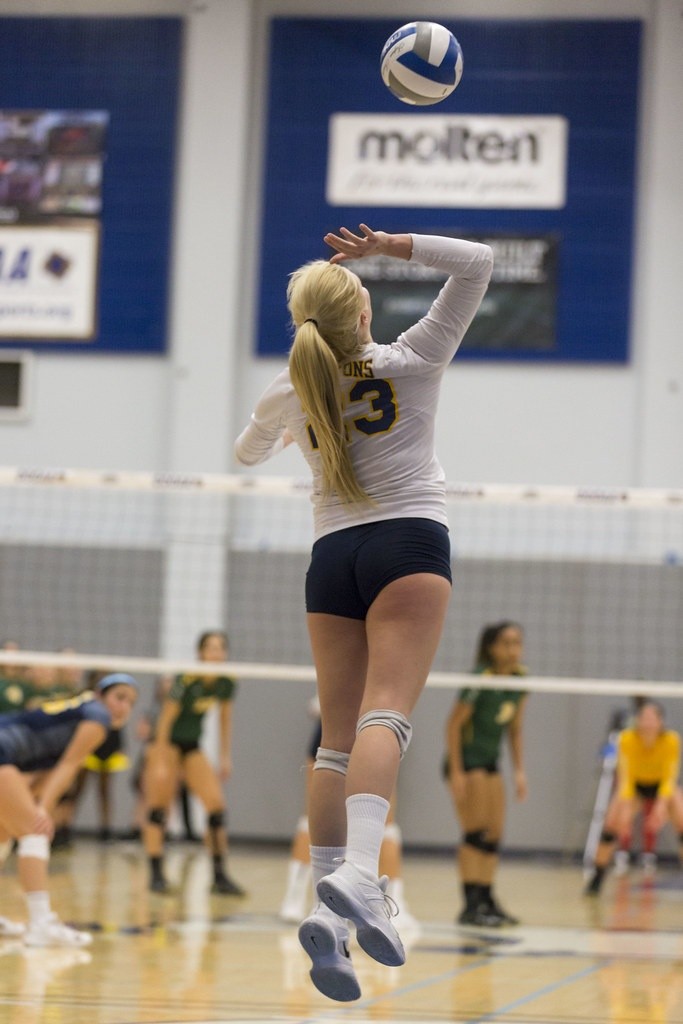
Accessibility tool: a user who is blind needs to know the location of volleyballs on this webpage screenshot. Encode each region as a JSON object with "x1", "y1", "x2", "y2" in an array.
[{"x1": 378, "y1": 20, "x2": 464, "y2": 107}]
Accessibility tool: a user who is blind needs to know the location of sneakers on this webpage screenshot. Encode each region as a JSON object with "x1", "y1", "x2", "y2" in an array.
[
  {"x1": 297, "y1": 908, "x2": 360, "y2": 1004},
  {"x1": 458, "y1": 879, "x2": 518, "y2": 931},
  {"x1": 211, "y1": 862, "x2": 247, "y2": 897},
  {"x1": 19, "y1": 892, "x2": 91, "y2": 950},
  {"x1": 147, "y1": 855, "x2": 173, "y2": 894},
  {"x1": 1, "y1": 916, "x2": 26, "y2": 936},
  {"x1": 316, "y1": 852, "x2": 408, "y2": 967}
]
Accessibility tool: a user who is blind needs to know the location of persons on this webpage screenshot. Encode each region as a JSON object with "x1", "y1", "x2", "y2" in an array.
[
  {"x1": 5, "y1": 636, "x2": 205, "y2": 854},
  {"x1": 230, "y1": 220, "x2": 495, "y2": 1003},
  {"x1": 131, "y1": 629, "x2": 248, "y2": 901},
  {"x1": 0, "y1": 671, "x2": 141, "y2": 950},
  {"x1": 583, "y1": 695, "x2": 683, "y2": 898},
  {"x1": 442, "y1": 618, "x2": 530, "y2": 933}
]
